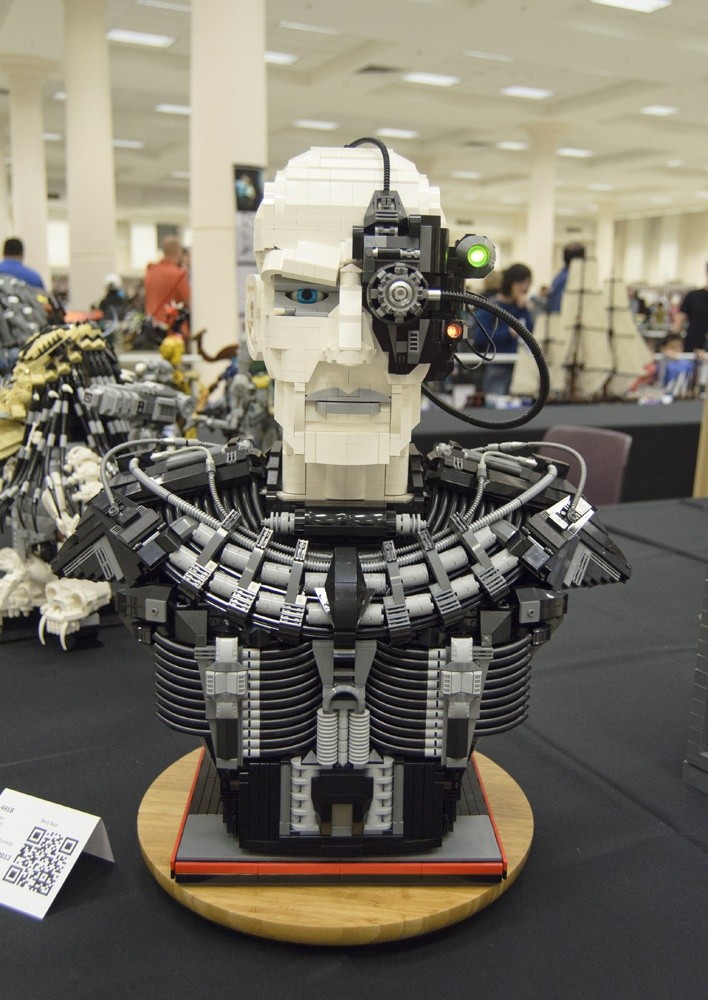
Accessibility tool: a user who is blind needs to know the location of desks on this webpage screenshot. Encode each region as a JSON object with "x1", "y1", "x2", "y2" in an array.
[
  {"x1": 416, "y1": 400, "x2": 705, "y2": 502},
  {"x1": 0, "y1": 502, "x2": 708, "y2": 1000}
]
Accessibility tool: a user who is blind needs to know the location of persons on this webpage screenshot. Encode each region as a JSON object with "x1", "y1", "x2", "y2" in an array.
[
  {"x1": 0, "y1": 237, "x2": 45, "y2": 378},
  {"x1": 674, "y1": 258, "x2": 708, "y2": 356},
  {"x1": 146, "y1": 230, "x2": 190, "y2": 335},
  {"x1": 40, "y1": 137, "x2": 632, "y2": 886},
  {"x1": 470, "y1": 263, "x2": 534, "y2": 353},
  {"x1": 98, "y1": 271, "x2": 129, "y2": 316},
  {"x1": 529, "y1": 240, "x2": 653, "y2": 325}
]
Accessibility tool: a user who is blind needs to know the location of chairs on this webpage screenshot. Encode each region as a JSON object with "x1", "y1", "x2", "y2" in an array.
[{"x1": 541, "y1": 424, "x2": 633, "y2": 503}]
